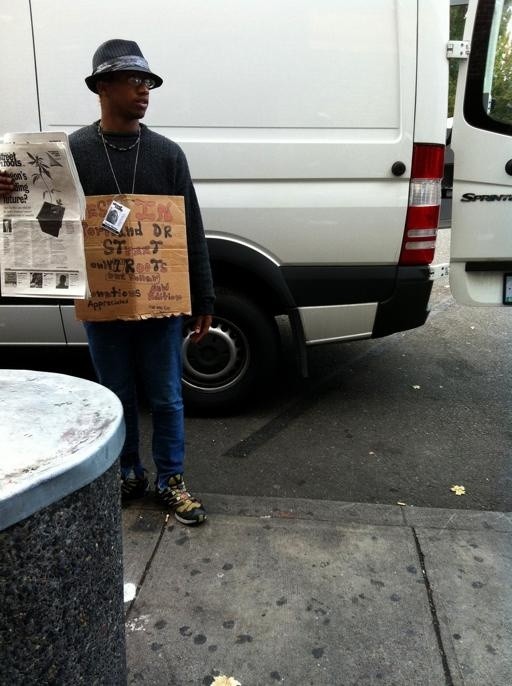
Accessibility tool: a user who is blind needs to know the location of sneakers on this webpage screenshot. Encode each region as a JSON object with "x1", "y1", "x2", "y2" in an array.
[
  {"x1": 155, "y1": 473, "x2": 207, "y2": 526},
  {"x1": 120, "y1": 470, "x2": 148, "y2": 499}
]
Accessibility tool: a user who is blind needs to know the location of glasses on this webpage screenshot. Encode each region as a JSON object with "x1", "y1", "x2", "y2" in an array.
[{"x1": 125, "y1": 73, "x2": 155, "y2": 89}]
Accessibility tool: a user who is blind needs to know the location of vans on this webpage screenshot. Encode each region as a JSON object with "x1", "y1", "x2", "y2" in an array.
[{"x1": 2, "y1": 0, "x2": 512, "y2": 416}]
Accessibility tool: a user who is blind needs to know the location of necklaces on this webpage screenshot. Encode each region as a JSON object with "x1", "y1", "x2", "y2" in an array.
[{"x1": 97, "y1": 125, "x2": 141, "y2": 151}]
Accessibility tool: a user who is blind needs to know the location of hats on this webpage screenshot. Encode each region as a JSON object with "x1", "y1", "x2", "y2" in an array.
[{"x1": 85, "y1": 39, "x2": 163, "y2": 94}]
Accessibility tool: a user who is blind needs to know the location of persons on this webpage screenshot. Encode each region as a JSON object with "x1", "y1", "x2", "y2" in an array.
[
  {"x1": 0, "y1": 38, "x2": 216, "y2": 525},
  {"x1": 107, "y1": 210, "x2": 118, "y2": 225}
]
